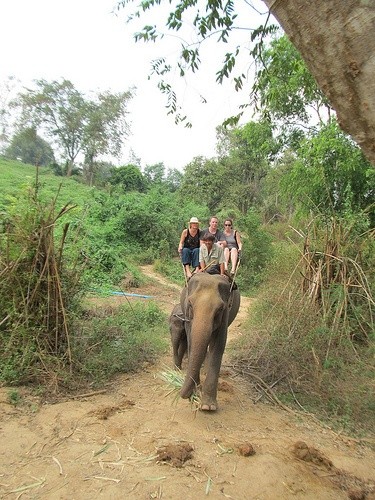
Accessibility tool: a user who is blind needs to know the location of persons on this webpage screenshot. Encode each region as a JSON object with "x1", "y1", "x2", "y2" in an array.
[
  {"x1": 199, "y1": 232, "x2": 227, "y2": 277},
  {"x1": 199, "y1": 216, "x2": 228, "y2": 248},
  {"x1": 178, "y1": 217, "x2": 203, "y2": 278},
  {"x1": 217, "y1": 218, "x2": 242, "y2": 275}
]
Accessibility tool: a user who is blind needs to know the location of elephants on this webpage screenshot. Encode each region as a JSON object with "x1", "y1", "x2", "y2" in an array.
[
  {"x1": 179, "y1": 272, "x2": 240, "y2": 411},
  {"x1": 170, "y1": 304, "x2": 188, "y2": 368}
]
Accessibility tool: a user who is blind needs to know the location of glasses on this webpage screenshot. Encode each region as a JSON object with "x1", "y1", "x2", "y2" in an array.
[
  {"x1": 225, "y1": 224, "x2": 231, "y2": 226},
  {"x1": 211, "y1": 222, "x2": 218, "y2": 223}
]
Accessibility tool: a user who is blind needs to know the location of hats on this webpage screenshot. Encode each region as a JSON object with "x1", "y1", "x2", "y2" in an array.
[{"x1": 188, "y1": 217, "x2": 199, "y2": 223}]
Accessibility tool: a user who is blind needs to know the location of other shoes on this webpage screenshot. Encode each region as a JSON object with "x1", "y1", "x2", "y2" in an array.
[
  {"x1": 230, "y1": 270, "x2": 235, "y2": 275},
  {"x1": 224, "y1": 268, "x2": 229, "y2": 272}
]
